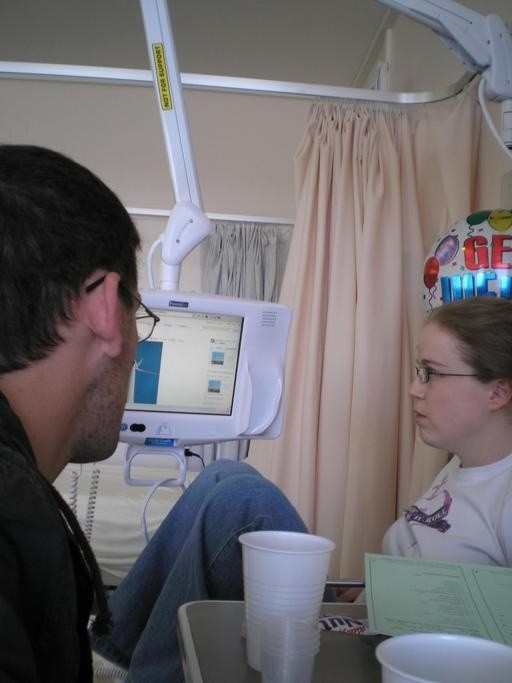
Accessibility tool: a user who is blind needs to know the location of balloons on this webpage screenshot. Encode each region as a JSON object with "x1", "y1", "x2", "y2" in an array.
[{"x1": 420, "y1": 209, "x2": 512, "y2": 316}]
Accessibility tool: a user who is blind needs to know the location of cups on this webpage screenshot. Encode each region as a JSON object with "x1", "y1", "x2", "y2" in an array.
[
  {"x1": 260, "y1": 616, "x2": 320, "y2": 682},
  {"x1": 237, "y1": 531, "x2": 335, "y2": 670}
]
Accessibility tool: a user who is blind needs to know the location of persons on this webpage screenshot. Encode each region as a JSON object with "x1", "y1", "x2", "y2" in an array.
[
  {"x1": 90, "y1": 298, "x2": 512, "y2": 682},
  {"x1": 0, "y1": 144, "x2": 143, "y2": 683}
]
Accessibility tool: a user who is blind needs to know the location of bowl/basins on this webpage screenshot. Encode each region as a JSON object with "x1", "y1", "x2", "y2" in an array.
[{"x1": 375, "y1": 632, "x2": 512, "y2": 681}]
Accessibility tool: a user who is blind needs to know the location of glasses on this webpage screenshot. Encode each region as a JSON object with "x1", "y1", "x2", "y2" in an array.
[
  {"x1": 85, "y1": 272, "x2": 160, "y2": 344},
  {"x1": 413, "y1": 365, "x2": 478, "y2": 384}
]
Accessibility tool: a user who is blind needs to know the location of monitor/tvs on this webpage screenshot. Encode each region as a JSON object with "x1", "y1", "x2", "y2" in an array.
[{"x1": 117, "y1": 288, "x2": 295, "y2": 448}]
